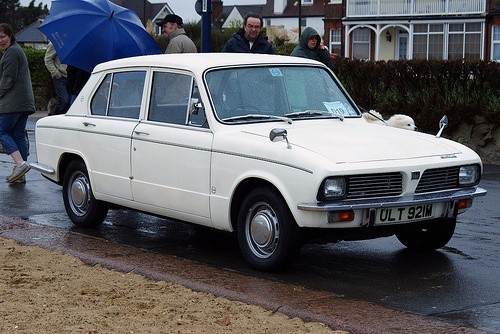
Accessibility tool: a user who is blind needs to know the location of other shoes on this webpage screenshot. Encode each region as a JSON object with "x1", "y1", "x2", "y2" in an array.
[{"x1": 6, "y1": 162, "x2": 31, "y2": 184}]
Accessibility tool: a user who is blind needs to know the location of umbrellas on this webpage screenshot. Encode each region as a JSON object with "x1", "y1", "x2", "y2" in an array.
[{"x1": 37, "y1": 0, "x2": 163, "y2": 74}]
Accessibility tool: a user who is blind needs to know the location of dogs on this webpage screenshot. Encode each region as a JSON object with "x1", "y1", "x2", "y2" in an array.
[{"x1": 361, "y1": 109, "x2": 418, "y2": 131}]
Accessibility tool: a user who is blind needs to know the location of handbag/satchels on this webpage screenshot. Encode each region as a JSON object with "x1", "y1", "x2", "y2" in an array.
[{"x1": 0, "y1": 128, "x2": 30, "y2": 156}]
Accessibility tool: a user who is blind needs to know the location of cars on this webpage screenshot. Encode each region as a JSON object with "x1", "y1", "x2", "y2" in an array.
[{"x1": 29, "y1": 53, "x2": 488, "y2": 275}]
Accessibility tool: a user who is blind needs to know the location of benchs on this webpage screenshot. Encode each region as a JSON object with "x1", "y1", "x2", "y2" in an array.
[{"x1": 98, "y1": 102, "x2": 190, "y2": 126}]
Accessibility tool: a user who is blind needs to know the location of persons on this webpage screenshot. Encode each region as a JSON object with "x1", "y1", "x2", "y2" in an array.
[
  {"x1": 63, "y1": 63, "x2": 93, "y2": 107},
  {"x1": 213, "y1": 9, "x2": 279, "y2": 115},
  {"x1": 0, "y1": 23, "x2": 35, "y2": 184},
  {"x1": 291, "y1": 25, "x2": 334, "y2": 76},
  {"x1": 43, "y1": 38, "x2": 70, "y2": 117},
  {"x1": 156, "y1": 14, "x2": 199, "y2": 105}
]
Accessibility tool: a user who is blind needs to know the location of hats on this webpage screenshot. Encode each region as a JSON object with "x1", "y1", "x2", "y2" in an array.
[{"x1": 156, "y1": 14, "x2": 183, "y2": 26}]
